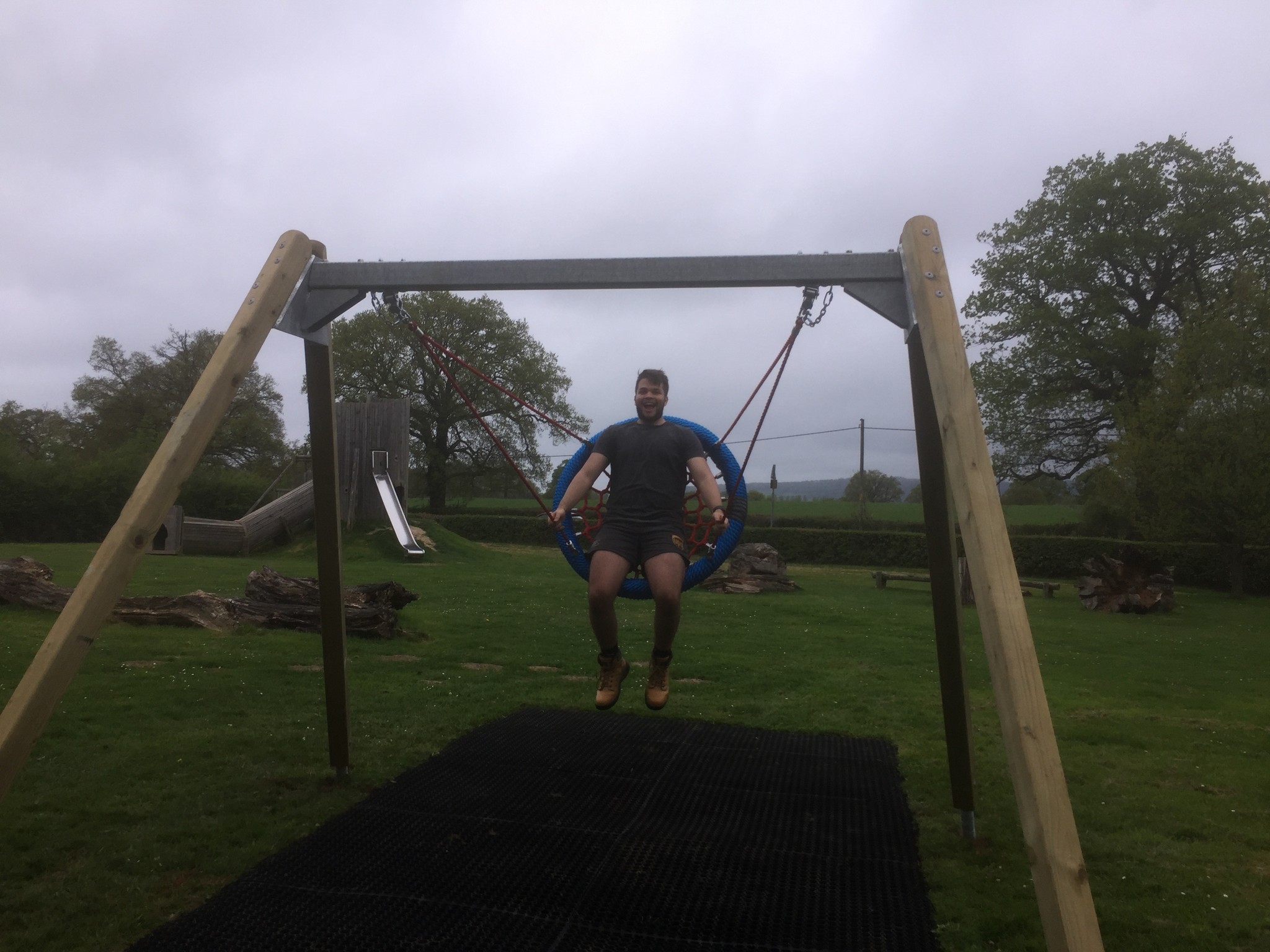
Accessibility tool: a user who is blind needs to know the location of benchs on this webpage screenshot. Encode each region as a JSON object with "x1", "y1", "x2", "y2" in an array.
[{"x1": 871, "y1": 568, "x2": 1066, "y2": 600}]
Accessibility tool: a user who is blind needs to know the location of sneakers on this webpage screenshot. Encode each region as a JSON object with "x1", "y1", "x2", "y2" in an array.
[
  {"x1": 644, "y1": 649, "x2": 673, "y2": 710},
  {"x1": 596, "y1": 650, "x2": 629, "y2": 711}
]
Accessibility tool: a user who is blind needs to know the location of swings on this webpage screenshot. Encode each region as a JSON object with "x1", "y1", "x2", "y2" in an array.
[{"x1": 371, "y1": 284, "x2": 833, "y2": 600}]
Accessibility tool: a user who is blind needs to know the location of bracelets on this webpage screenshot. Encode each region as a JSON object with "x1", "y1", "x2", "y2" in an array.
[{"x1": 712, "y1": 505, "x2": 726, "y2": 514}]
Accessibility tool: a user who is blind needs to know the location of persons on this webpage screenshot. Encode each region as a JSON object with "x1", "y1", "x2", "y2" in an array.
[{"x1": 548, "y1": 368, "x2": 729, "y2": 711}]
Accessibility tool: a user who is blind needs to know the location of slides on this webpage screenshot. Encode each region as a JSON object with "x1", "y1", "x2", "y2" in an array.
[{"x1": 372, "y1": 470, "x2": 425, "y2": 554}]
